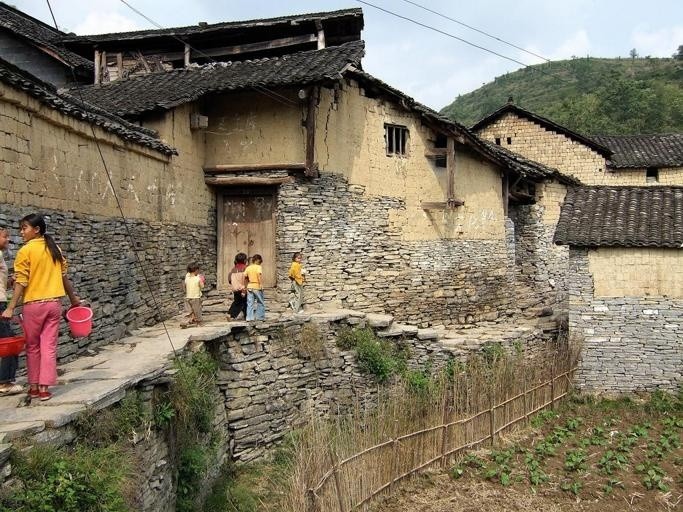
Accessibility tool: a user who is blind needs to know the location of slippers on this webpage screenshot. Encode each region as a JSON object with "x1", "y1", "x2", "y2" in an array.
[{"x1": 0, "y1": 384, "x2": 26, "y2": 397}]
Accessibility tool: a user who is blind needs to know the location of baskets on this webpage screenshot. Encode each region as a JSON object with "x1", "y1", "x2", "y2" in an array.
[{"x1": 0, "y1": 314, "x2": 27, "y2": 357}]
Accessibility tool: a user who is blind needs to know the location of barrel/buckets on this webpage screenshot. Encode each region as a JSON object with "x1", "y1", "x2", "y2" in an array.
[{"x1": 66, "y1": 299, "x2": 93, "y2": 337}]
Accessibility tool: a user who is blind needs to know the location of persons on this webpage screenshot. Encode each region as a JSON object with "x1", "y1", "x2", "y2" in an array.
[
  {"x1": 2, "y1": 214, "x2": 82, "y2": 401},
  {"x1": 0, "y1": 225, "x2": 24, "y2": 397},
  {"x1": 180, "y1": 252, "x2": 305, "y2": 329}
]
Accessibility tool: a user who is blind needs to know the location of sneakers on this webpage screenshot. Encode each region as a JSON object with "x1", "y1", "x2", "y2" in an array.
[
  {"x1": 28, "y1": 390, "x2": 39, "y2": 398},
  {"x1": 38, "y1": 392, "x2": 54, "y2": 401}
]
